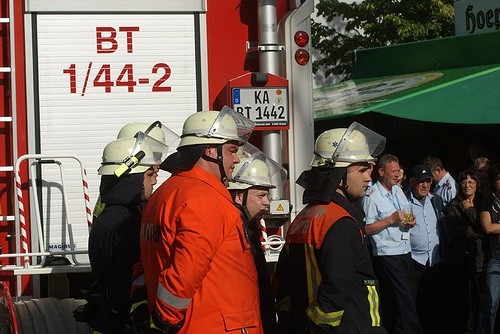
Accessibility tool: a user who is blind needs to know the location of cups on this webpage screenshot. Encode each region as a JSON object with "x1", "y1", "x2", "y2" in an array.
[{"x1": 400, "y1": 204, "x2": 413, "y2": 227}]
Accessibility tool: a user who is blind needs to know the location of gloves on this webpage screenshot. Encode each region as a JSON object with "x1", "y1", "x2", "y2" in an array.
[
  {"x1": 86, "y1": 286, "x2": 128, "y2": 334},
  {"x1": 143, "y1": 311, "x2": 178, "y2": 334},
  {"x1": 129, "y1": 296, "x2": 150, "y2": 334},
  {"x1": 72, "y1": 283, "x2": 114, "y2": 321}
]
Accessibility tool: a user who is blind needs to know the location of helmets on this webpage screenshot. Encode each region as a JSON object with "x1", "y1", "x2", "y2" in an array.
[
  {"x1": 176, "y1": 110, "x2": 245, "y2": 149},
  {"x1": 309, "y1": 128, "x2": 378, "y2": 167},
  {"x1": 116, "y1": 122, "x2": 165, "y2": 145},
  {"x1": 96, "y1": 139, "x2": 157, "y2": 175},
  {"x1": 226, "y1": 158, "x2": 276, "y2": 189}
]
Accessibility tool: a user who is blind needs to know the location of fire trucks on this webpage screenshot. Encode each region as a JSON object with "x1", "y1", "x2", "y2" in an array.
[{"x1": 0, "y1": 0, "x2": 315, "y2": 327}]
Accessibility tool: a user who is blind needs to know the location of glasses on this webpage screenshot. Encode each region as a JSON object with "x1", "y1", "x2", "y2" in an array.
[
  {"x1": 460, "y1": 180, "x2": 477, "y2": 185},
  {"x1": 416, "y1": 178, "x2": 432, "y2": 183},
  {"x1": 477, "y1": 169, "x2": 490, "y2": 174}
]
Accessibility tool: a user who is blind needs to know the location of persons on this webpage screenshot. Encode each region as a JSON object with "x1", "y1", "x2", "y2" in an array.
[
  {"x1": 130, "y1": 105, "x2": 265, "y2": 334},
  {"x1": 72, "y1": 121, "x2": 287, "y2": 334},
  {"x1": 361, "y1": 154, "x2": 500, "y2": 334},
  {"x1": 272, "y1": 121, "x2": 389, "y2": 334}
]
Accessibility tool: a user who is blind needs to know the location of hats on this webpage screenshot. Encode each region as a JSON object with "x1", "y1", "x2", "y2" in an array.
[{"x1": 412, "y1": 165, "x2": 434, "y2": 181}]
